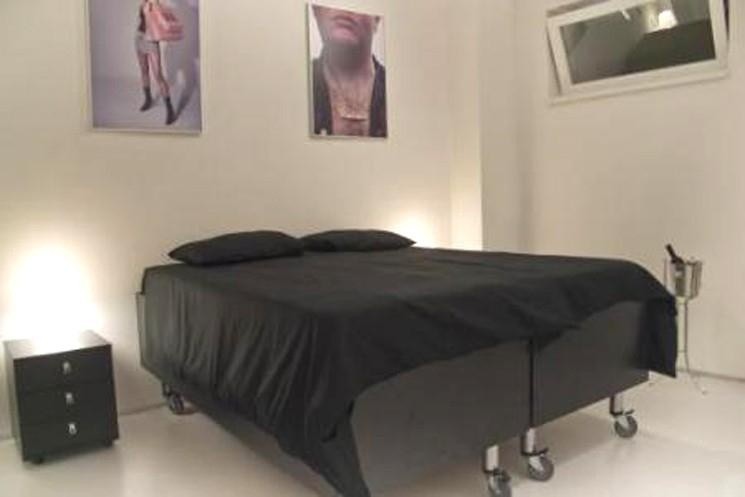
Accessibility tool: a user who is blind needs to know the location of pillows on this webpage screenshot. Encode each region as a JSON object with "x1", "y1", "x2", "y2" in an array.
[{"x1": 169, "y1": 227, "x2": 418, "y2": 269}]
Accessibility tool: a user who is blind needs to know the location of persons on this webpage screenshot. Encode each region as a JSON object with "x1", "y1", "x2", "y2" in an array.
[
  {"x1": 310, "y1": 3, "x2": 388, "y2": 136},
  {"x1": 132, "y1": 0, "x2": 178, "y2": 124}
]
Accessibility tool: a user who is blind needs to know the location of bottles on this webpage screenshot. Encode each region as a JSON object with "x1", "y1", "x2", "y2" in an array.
[{"x1": 666, "y1": 242, "x2": 684, "y2": 266}]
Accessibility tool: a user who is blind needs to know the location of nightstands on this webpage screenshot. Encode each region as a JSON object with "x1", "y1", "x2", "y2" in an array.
[{"x1": 1, "y1": 330, "x2": 122, "y2": 463}]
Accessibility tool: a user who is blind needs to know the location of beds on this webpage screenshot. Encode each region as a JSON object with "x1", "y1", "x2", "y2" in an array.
[{"x1": 134, "y1": 244, "x2": 652, "y2": 494}]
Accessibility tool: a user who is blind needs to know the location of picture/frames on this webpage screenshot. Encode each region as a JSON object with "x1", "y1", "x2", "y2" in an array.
[{"x1": 84, "y1": 0, "x2": 206, "y2": 136}]
